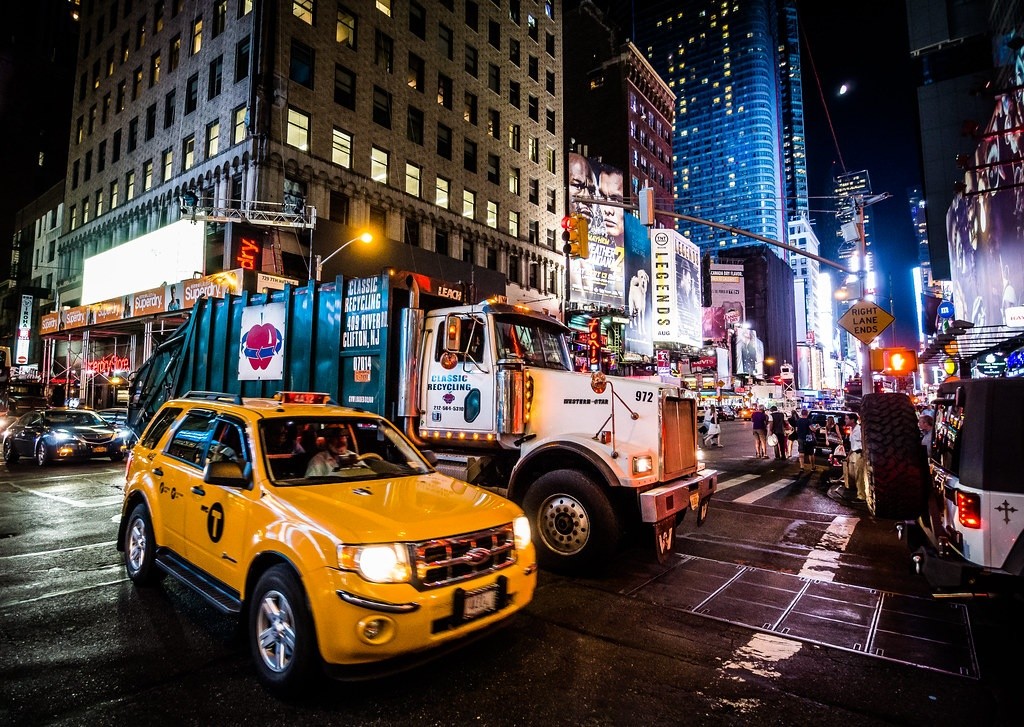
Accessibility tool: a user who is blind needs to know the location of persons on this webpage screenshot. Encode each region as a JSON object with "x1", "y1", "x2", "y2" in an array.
[
  {"x1": 122, "y1": 295, "x2": 131, "y2": 319},
  {"x1": 58, "y1": 312, "x2": 64, "y2": 330},
  {"x1": 304, "y1": 427, "x2": 369, "y2": 477},
  {"x1": 168, "y1": 285, "x2": 180, "y2": 312},
  {"x1": 568, "y1": 152, "x2": 624, "y2": 305},
  {"x1": 87, "y1": 308, "x2": 94, "y2": 324},
  {"x1": 702, "y1": 373, "x2": 936, "y2": 503},
  {"x1": 741, "y1": 330, "x2": 756, "y2": 375}
]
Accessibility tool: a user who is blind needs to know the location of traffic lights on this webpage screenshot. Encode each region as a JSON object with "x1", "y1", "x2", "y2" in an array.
[
  {"x1": 562, "y1": 215, "x2": 589, "y2": 259},
  {"x1": 884, "y1": 350, "x2": 918, "y2": 373}
]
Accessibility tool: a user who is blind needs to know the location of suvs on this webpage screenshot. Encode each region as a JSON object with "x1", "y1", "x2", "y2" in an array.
[
  {"x1": 116, "y1": 390, "x2": 539, "y2": 683},
  {"x1": 860, "y1": 377, "x2": 1024, "y2": 598}
]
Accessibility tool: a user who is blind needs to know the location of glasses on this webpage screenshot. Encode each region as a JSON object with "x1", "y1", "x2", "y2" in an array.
[{"x1": 333, "y1": 435, "x2": 349, "y2": 439}]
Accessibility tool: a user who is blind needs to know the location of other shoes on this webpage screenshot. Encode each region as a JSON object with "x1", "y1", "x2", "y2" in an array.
[
  {"x1": 704, "y1": 441, "x2": 709, "y2": 448},
  {"x1": 712, "y1": 442, "x2": 718, "y2": 445},
  {"x1": 833, "y1": 460, "x2": 843, "y2": 467},
  {"x1": 799, "y1": 468, "x2": 805, "y2": 472},
  {"x1": 702, "y1": 438, "x2": 706, "y2": 446},
  {"x1": 852, "y1": 498, "x2": 867, "y2": 504},
  {"x1": 811, "y1": 466, "x2": 817, "y2": 472},
  {"x1": 756, "y1": 455, "x2": 761, "y2": 459},
  {"x1": 718, "y1": 445, "x2": 726, "y2": 448},
  {"x1": 762, "y1": 456, "x2": 770, "y2": 459},
  {"x1": 827, "y1": 457, "x2": 833, "y2": 465}
]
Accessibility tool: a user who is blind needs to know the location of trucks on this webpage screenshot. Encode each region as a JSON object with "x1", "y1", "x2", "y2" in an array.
[{"x1": 126, "y1": 267, "x2": 719, "y2": 579}]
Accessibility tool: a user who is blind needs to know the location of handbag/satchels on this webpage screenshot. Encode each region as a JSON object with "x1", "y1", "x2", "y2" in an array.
[
  {"x1": 767, "y1": 433, "x2": 778, "y2": 447},
  {"x1": 834, "y1": 444, "x2": 847, "y2": 458}
]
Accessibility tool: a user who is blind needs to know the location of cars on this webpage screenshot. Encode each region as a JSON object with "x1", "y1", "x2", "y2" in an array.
[
  {"x1": 696, "y1": 406, "x2": 758, "y2": 422},
  {"x1": 2, "y1": 409, "x2": 127, "y2": 469},
  {"x1": 805, "y1": 409, "x2": 861, "y2": 456}
]
